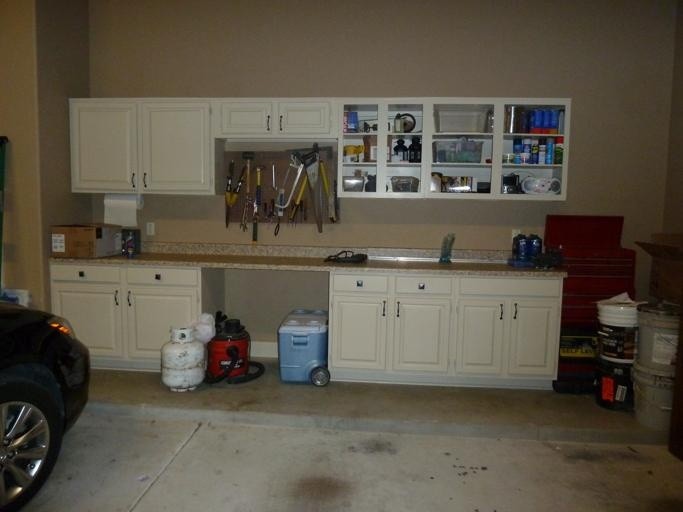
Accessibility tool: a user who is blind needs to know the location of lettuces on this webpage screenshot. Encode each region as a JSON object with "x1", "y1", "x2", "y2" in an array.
[{"x1": 243, "y1": 151, "x2": 255, "y2": 193}]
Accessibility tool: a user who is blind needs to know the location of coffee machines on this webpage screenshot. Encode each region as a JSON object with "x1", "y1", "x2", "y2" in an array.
[{"x1": 225, "y1": 160, "x2": 248, "y2": 228}]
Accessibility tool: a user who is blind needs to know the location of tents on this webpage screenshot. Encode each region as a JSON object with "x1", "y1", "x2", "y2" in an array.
[{"x1": 395, "y1": 111, "x2": 416, "y2": 134}]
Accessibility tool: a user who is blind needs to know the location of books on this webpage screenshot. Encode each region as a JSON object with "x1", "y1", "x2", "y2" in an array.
[
  {"x1": 276, "y1": 309, "x2": 328, "y2": 384},
  {"x1": 633, "y1": 232, "x2": 683, "y2": 305},
  {"x1": 50, "y1": 219, "x2": 122, "y2": 259}
]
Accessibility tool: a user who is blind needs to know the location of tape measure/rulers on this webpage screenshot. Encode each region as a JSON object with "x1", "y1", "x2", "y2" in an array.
[{"x1": 264, "y1": 199, "x2": 274, "y2": 225}]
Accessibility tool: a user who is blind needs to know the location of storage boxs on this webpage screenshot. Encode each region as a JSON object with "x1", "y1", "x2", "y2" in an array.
[
  {"x1": 50, "y1": 219, "x2": 122, "y2": 259},
  {"x1": 276, "y1": 309, "x2": 328, "y2": 384},
  {"x1": 633, "y1": 232, "x2": 683, "y2": 305}
]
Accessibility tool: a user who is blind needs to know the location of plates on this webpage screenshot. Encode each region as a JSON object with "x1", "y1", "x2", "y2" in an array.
[
  {"x1": 327, "y1": 270, "x2": 564, "y2": 392},
  {"x1": 545, "y1": 246, "x2": 636, "y2": 375},
  {"x1": 46, "y1": 261, "x2": 226, "y2": 373}
]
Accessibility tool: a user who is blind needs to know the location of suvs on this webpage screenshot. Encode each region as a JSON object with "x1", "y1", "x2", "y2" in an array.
[
  {"x1": 631, "y1": 360, "x2": 683, "y2": 432},
  {"x1": 635, "y1": 306, "x2": 682, "y2": 371},
  {"x1": 594, "y1": 359, "x2": 634, "y2": 413},
  {"x1": 596, "y1": 301, "x2": 641, "y2": 363}
]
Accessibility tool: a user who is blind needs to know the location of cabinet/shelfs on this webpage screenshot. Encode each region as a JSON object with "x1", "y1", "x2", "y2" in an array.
[
  {"x1": 513, "y1": 233, "x2": 542, "y2": 261},
  {"x1": 46, "y1": 261, "x2": 226, "y2": 373},
  {"x1": 70, "y1": 98, "x2": 227, "y2": 195},
  {"x1": 337, "y1": 97, "x2": 428, "y2": 199},
  {"x1": 127, "y1": 232, "x2": 135, "y2": 259},
  {"x1": 213, "y1": 97, "x2": 342, "y2": 141},
  {"x1": 394, "y1": 138, "x2": 421, "y2": 163},
  {"x1": 427, "y1": 98, "x2": 570, "y2": 200},
  {"x1": 545, "y1": 246, "x2": 636, "y2": 375},
  {"x1": 502, "y1": 105, "x2": 564, "y2": 164},
  {"x1": 327, "y1": 270, "x2": 564, "y2": 392}
]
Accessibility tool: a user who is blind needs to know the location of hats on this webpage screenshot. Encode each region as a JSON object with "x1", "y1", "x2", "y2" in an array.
[{"x1": 1, "y1": 301, "x2": 91, "y2": 511}]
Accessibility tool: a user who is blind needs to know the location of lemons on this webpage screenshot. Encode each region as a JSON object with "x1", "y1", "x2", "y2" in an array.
[{"x1": 521, "y1": 177, "x2": 551, "y2": 195}]
